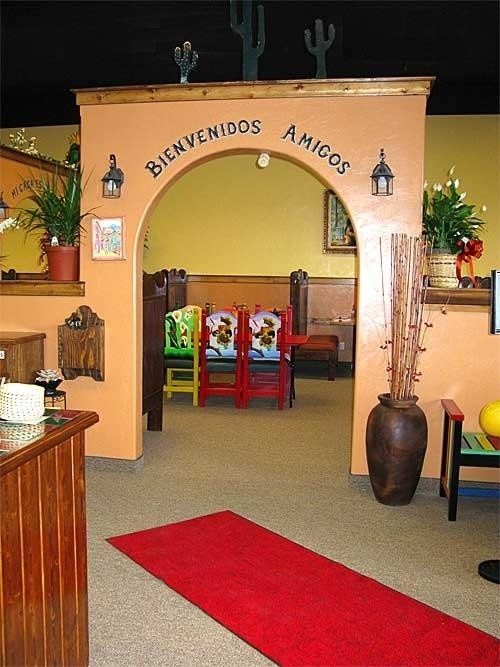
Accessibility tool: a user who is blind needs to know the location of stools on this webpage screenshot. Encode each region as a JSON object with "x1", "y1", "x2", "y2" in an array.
[{"x1": 439, "y1": 397, "x2": 500, "y2": 522}]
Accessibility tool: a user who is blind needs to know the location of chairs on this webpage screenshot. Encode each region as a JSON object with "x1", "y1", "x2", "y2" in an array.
[
  {"x1": 287, "y1": 270, "x2": 338, "y2": 381},
  {"x1": 162, "y1": 301, "x2": 292, "y2": 411}
]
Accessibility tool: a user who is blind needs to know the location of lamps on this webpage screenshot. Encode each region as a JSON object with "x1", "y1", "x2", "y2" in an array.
[
  {"x1": 369, "y1": 147, "x2": 395, "y2": 197},
  {"x1": 100, "y1": 154, "x2": 124, "y2": 198}
]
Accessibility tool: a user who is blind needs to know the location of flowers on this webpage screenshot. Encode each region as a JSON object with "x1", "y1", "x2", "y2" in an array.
[{"x1": 422, "y1": 167, "x2": 488, "y2": 258}]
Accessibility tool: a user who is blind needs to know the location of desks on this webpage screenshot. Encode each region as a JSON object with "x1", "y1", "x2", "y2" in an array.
[
  {"x1": 0, "y1": 330, "x2": 47, "y2": 386},
  {"x1": 310, "y1": 317, "x2": 355, "y2": 378},
  {"x1": 44, "y1": 391, "x2": 66, "y2": 410},
  {"x1": 0, "y1": 406, "x2": 100, "y2": 667},
  {"x1": 168, "y1": 330, "x2": 310, "y2": 409}
]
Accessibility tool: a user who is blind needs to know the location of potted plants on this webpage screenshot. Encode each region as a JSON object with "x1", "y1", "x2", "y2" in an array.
[{"x1": 12, "y1": 153, "x2": 103, "y2": 285}]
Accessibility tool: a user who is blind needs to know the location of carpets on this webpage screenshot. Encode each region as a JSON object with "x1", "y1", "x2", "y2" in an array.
[{"x1": 107, "y1": 507, "x2": 499, "y2": 667}]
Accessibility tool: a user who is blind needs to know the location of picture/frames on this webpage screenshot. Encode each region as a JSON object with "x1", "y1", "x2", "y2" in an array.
[
  {"x1": 491, "y1": 269, "x2": 500, "y2": 335},
  {"x1": 90, "y1": 216, "x2": 125, "y2": 260},
  {"x1": 322, "y1": 188, "x2": 358, "y2": 254}
]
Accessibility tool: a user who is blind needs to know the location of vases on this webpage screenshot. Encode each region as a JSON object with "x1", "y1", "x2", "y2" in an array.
[{"x1": 364, "y1": 392, "x2": 428, "y2": 507}]
